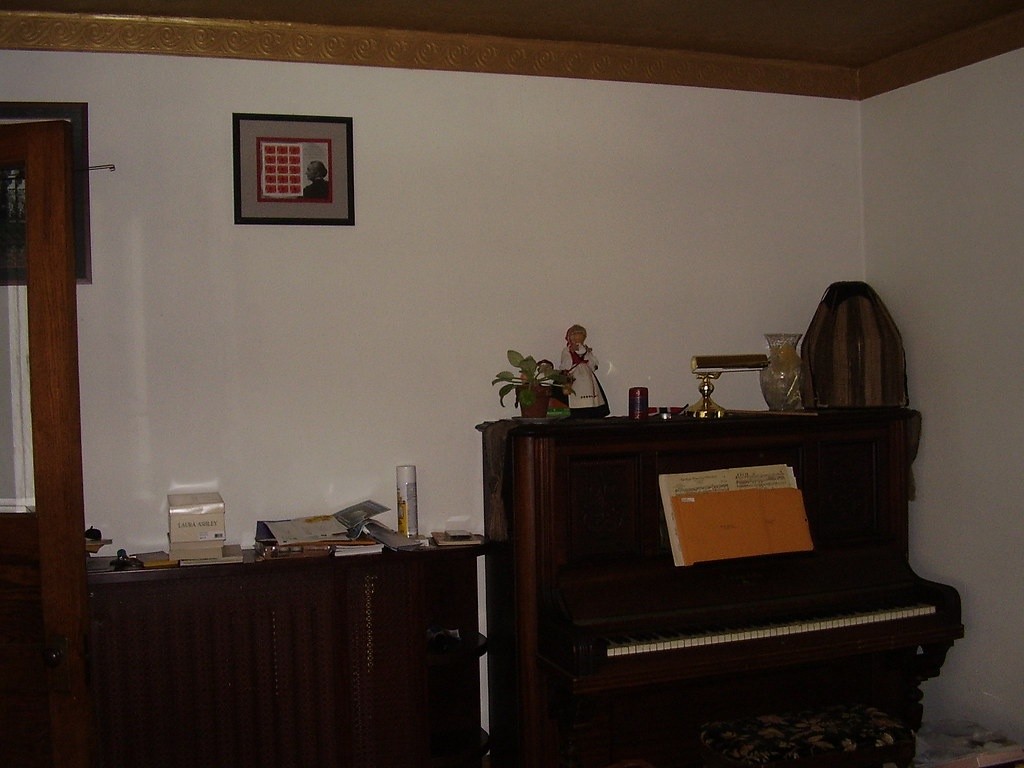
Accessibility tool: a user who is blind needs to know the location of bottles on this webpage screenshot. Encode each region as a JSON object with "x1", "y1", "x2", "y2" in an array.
[
  {"x1": 396, "y1": 466, "x2": 418, "y2": 539},
  {"x1": 759, "y1": 334, "x2": 805, "y2": 411}
]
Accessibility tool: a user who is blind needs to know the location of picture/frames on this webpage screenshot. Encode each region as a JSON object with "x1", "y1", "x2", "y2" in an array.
[{"x1": 232, "y1": 112, "x2": 356, "y2": 226}]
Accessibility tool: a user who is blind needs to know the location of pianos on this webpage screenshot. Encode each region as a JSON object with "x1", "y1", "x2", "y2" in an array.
[{"x1": 473, "y1": 404, "x2": 966, "y2": 768}]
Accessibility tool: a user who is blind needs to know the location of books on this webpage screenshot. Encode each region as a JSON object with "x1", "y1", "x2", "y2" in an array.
[
  {"x1": 431, "y1": 532, "x2": 481, "y2": 545},
  {"x1": 331, "y1": 544, "x2": 385, "y2": 557},
  {"x1": 129, "y1": 532, "x2": 244, "y2": 567},
  {"x1": 657, "y1": 463, "x2": 814, "y2": 566}
]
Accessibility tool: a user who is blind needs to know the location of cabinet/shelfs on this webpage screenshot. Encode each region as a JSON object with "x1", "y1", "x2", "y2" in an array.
[{"x1": 85, "y1": 546, "x2": 496, "y2": 768}]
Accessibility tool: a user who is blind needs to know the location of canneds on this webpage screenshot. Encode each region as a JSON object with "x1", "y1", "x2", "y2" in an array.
[{"x1": 629, "y1": 387, "x2": 648, "y2": 421}]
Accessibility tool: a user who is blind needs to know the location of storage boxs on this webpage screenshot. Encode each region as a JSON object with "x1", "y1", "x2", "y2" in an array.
[{"x1": 164, "y1": 492, "x2": 226, "y2": 561}]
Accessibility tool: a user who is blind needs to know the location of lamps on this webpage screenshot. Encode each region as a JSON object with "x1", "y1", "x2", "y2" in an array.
[{"x1": 683, "y1": 354, "x2": 771, "y2": 419}]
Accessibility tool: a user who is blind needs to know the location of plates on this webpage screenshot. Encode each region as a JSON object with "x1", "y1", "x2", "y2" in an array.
[{"x1": 513, "y1": 416, "x2": 557, "y2": 425}]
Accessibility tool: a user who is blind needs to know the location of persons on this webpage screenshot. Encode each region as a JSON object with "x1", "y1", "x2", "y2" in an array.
[
  {"x1": 559, "y1": 324, "x2": 610, "y2": 419},
  {"x1": 298, "y1": 160, "x2": 330, "y2": 199}
]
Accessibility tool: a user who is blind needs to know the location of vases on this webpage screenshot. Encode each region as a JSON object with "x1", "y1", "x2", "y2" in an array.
[{"x1": 758, "y1": 333, "x2": 805, "y2": 414}]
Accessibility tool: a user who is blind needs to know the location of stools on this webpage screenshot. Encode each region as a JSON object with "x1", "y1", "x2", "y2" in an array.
[{"x1": 700, "y1": 697, "x2": 916, "y2": 768}]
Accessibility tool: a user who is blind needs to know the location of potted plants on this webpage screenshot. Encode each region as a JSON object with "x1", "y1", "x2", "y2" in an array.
[{"x1": 491, "y1": 349, "x2": 579, "y2": 420}]
